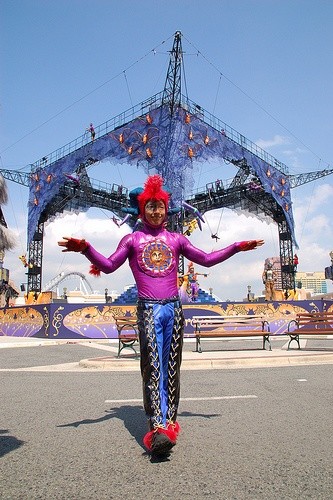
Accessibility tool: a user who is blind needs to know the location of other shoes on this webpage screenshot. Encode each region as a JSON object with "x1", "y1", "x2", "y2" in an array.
[{"x1": 149, "y1": 433, "x2": 175, "y2": 458}]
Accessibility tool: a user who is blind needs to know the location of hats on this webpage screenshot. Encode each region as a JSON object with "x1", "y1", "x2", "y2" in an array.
[{"x1": 137, "y1": 174, "x2": 168, "y2": 221}]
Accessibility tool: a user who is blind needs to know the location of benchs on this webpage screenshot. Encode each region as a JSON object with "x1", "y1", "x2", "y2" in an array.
[
  {"x1": 192, "y1": 313, "x2": 274, "y2": 354},
  {"x1": 112, "y1": 315, "x2": 139, "y2": 360},
  {"x1": 284, "y1": 312, "x2": 333, "y2": 351}
]
